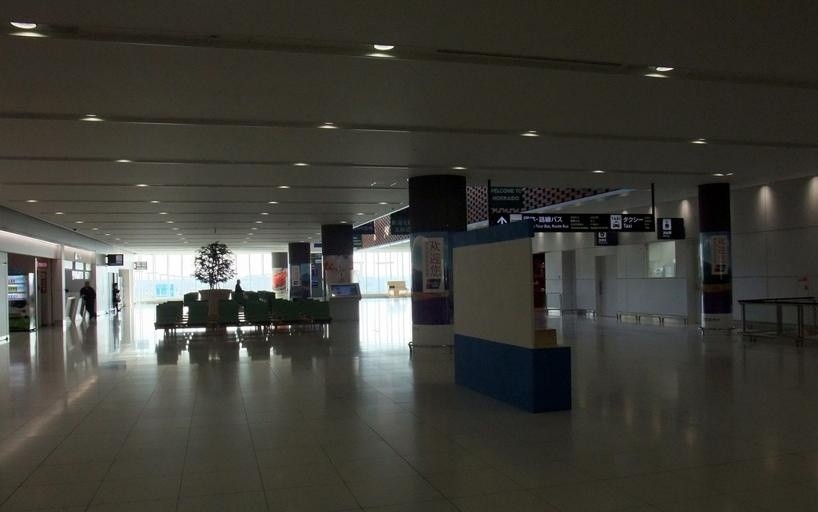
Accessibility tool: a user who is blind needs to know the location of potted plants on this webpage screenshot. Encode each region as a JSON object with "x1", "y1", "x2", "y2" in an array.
[{"x1": 192, "y1": 240, "x2": 239, "y2": 338}]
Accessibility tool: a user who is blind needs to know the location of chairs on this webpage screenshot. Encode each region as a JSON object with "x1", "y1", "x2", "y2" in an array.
[{"x1": 154, "y1": 290, "x2": 333, "y2": 336}]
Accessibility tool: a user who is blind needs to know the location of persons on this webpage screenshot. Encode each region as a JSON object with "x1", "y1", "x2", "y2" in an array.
[
  {"x1": 79, "y1": 281, "x2": 96, "y2": 320},
  {"x1": 235, "y1": 280, "x2": 244, "y2": 293},
  {"x1": 113, "y1": 283, "x2": 120, "y2": 312}
]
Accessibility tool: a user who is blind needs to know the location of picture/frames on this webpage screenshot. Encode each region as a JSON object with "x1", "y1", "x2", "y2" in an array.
[{"x1": 291, "y1": 265, "x2": 300, "y2": 287}]
